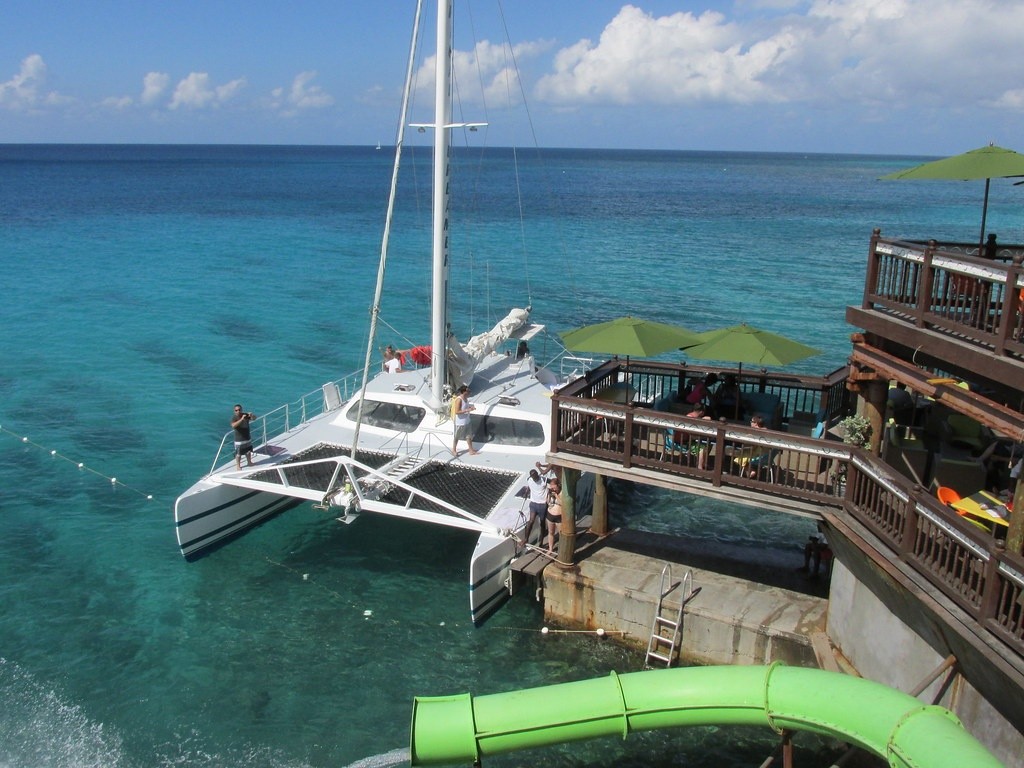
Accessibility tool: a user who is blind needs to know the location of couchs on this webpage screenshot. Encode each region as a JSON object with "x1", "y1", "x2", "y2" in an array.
[
  {"x1": 884, "y1": 393, "x2": 1020, "y2": 498},
  {"x1": 780, "y1": 409, "x2": 830, "y2": 473},
  {"x1": 738, "y1": 393, "x2": 785, "y2": 429},
  {"x1": 649, "y1": 392, "x2": 694, "y2": 446}
]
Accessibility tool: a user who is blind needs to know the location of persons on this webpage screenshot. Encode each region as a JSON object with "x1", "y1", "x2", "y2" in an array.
[
  {"x1": 888, "y1": 380, "x2": 914, "y2": 423},
  {"x1": 452, "y1": 385, "x2": 477, "y2": 456},
  {"x1": 978, "y1": 439, "x2": 1014, "y2": 492},
  {"x1": 733, "y1": 416, "x2": 776, "y2": 477},
  {"x1": 231, "y1": 405, "x2": 257, "y2": 470},
  {"x1": 798, "y1": 525, "x2": 829, "y2": 577},
  {"x1": 519, "y1": 462, "x2": 562, "y2": 558},
  {"x1": 379, "y1": 345, "x2": 411, "y2": 374},
  {"x1": 672, "y1": 402, "x2": 712, "y2": 470},
  {"x1": 686, "y1": 373, "x2": 737, "y2": 417},
  {"x1": 517, "y1": 342, "x2": 539, "y2": 379},
  {"x1": 1015, "y1": 288, "x2": 1024, "y2": 336}
]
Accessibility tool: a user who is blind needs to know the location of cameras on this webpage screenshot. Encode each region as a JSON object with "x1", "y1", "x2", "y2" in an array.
[{"x1": 244, "y1": 414, "x2": 251, "y2": 419}]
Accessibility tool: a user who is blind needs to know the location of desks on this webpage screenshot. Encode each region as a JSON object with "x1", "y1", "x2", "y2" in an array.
[
  {"x1": 593, "y1": 381, "x2": 637, "y2": 444},
  {"x1": 951, "y1": 489, "x2": 1012, "y2": 537}
]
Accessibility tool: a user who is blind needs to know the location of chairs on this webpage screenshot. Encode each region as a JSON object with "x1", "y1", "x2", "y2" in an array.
[
  {"x1": 937, "y1": 485, "x2": 971, "y2": 518},
  {"x1": 946, "y1": 273, "x2": 986, "y2": 319}
]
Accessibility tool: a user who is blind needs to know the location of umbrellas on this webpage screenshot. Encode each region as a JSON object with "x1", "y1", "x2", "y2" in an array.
[
  {"x1": 875, "y1": 142, "x2": 1024, "y2": 257},
  {"x1": 556, "y1": 316, "x2": 704, "y2": 405},
  {"x1": 680, "y1": 322, "x2": 824, "y2": 420}
]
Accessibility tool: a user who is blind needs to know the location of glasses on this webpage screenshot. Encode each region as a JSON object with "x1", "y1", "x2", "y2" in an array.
[
  {"x1": 235, "y1": 409, "x2": 241, "y2": 412},
  {"x1": 750, "y1": 420, "x2": 756, "y2": 423}
]
